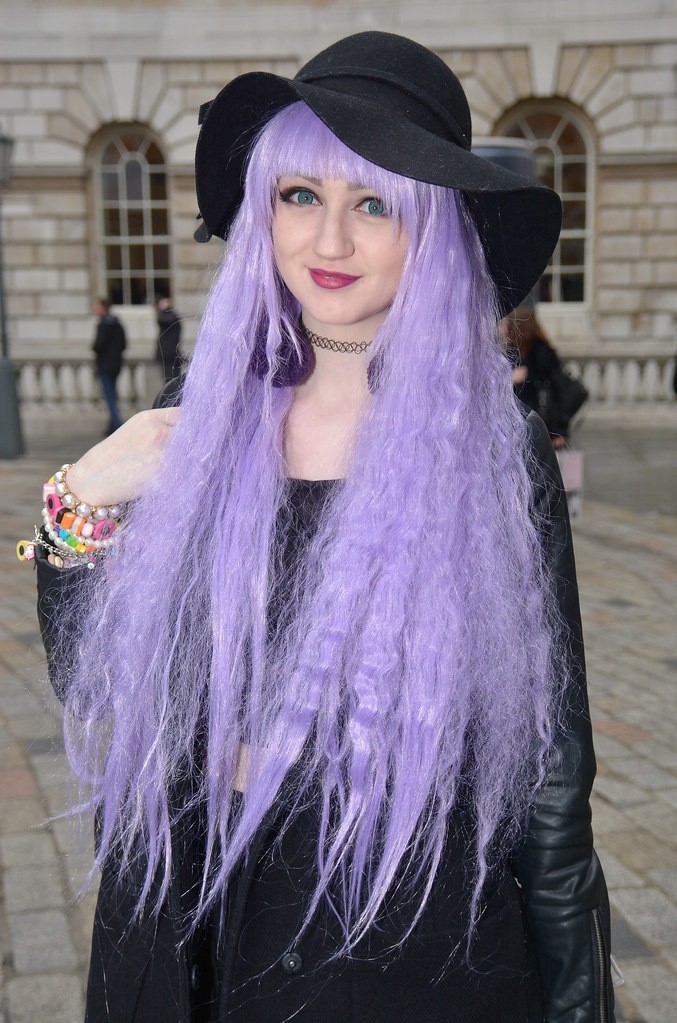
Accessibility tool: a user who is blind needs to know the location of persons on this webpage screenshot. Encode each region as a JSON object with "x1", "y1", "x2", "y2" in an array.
[
  {"x1": 500, "y1": 303, "x2": 589, "y2": 450},
  {"x1": 91, "y1": 297, "x2": 126, "y2": 436},
  {"x1": 34, "y1": 30, "x2": 616, "y2": 1023},
  {"x1": 155, "y1": 296, "x2": 182, "y2": 384}
]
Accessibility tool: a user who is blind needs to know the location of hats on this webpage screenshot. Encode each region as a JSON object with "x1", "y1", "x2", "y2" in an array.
[{"x1": 187, "y1": 31, "x2": 564, "y2": 328}]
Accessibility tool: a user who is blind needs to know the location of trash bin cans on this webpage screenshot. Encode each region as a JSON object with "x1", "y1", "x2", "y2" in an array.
[{"x1": 0, "y1": 360, "x2": 23, "y2": 460}]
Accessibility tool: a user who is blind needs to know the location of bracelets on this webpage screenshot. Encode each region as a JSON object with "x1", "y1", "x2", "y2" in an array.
[{"x1": 16, "y1": 462, "x2": 139, "y2": 570}]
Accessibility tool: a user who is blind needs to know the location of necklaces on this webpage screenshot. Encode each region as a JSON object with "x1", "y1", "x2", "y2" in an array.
[{"x1": 300, "y1": 323, "x2": 375, "y2": 354}]
